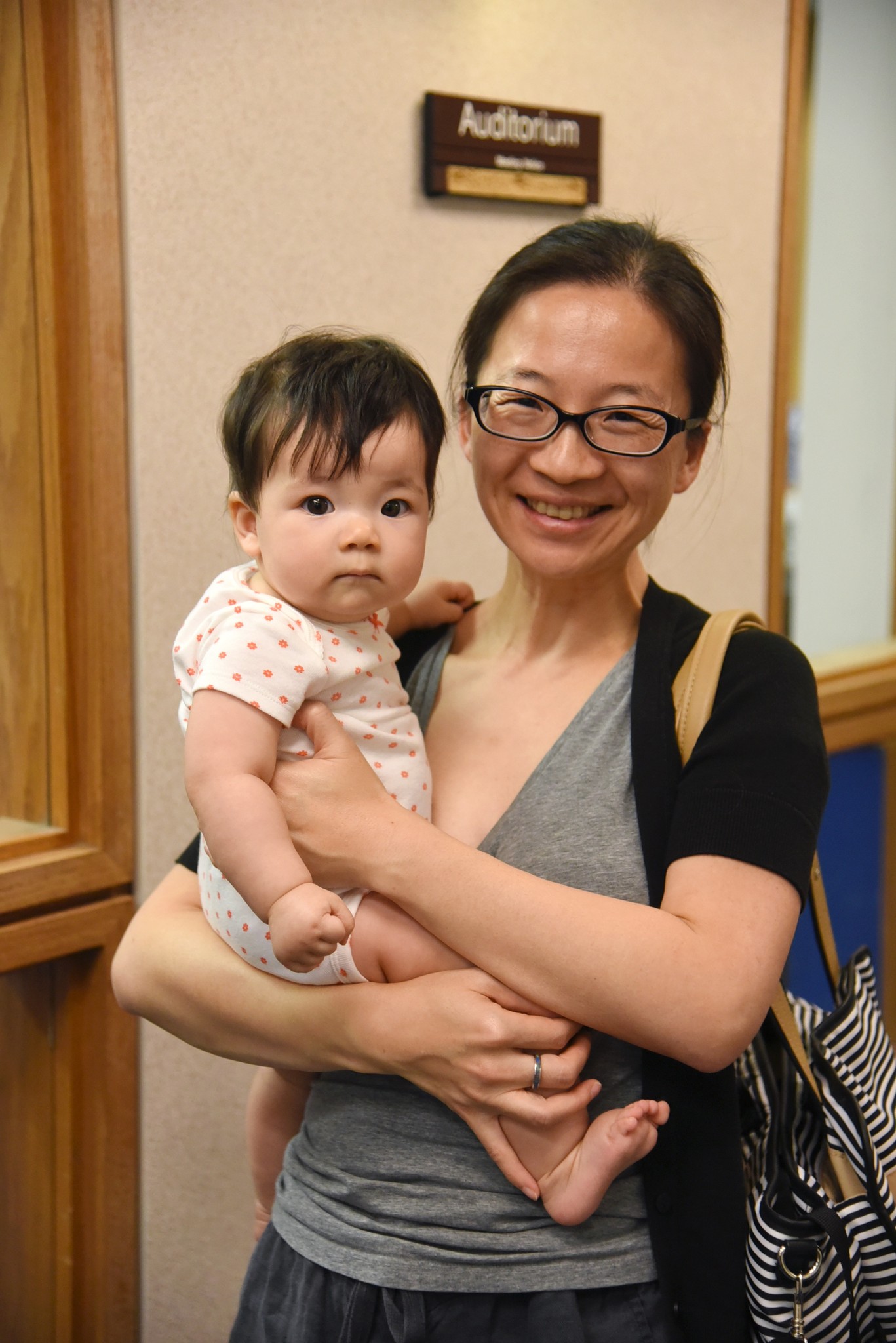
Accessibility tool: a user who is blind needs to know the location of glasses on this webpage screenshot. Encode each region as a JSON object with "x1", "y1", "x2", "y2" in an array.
[{"x1": 464, "y1": 385, "x2": 698, "y2": 459}]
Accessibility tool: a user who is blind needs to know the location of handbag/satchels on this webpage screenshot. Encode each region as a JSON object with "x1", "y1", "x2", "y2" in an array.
[{"x1": 670, "y1": 613, "x2": 896, "y2": 1343}]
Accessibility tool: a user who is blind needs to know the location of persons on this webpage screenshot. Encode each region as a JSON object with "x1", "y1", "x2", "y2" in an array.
[
  {"x1": 173, "y1": 332, "x2": 670, "y2": 1228},
  {"x1": 110, "y1": 213, "x2": 833, "y2": 1343}
]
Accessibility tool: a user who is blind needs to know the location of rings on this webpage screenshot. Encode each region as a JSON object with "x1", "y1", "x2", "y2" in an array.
[{"x1": 532, "y1": 1055, "x2": 542, "y2": 1089}]
